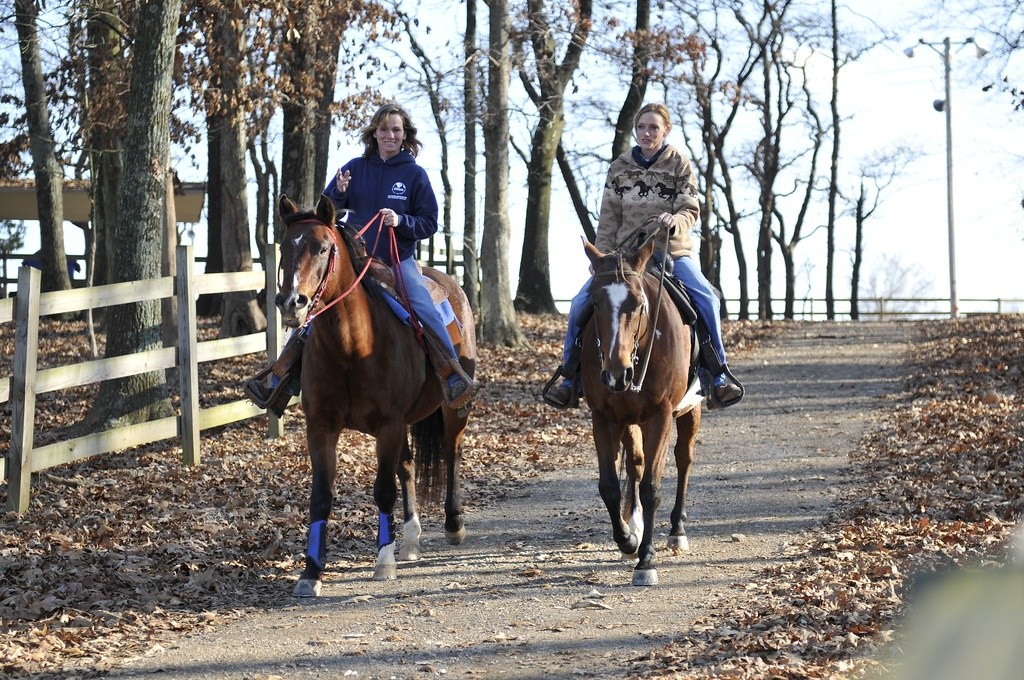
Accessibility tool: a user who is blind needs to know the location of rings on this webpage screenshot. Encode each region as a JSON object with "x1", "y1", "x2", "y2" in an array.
[{"x1": 385, "y1": 220, "x2": 389, "y2": 222}]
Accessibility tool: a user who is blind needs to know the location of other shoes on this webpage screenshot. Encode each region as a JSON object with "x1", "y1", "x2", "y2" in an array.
[
  {"x1": 716, "y1": 383, "x2": 740, "y2": 403},
  {"x1": 248, "y1": 378, "x2": 293, "y2": 418},
  {"x1": 547, "y1": 383, "x2": 579, "y2": 409},
  {"x1": 451, "y1": 381, "x2": 472, "y2": 419}
]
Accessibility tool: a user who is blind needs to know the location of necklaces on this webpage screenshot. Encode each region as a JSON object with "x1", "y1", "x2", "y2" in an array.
[{"x1": 640, "y1": 154, "x2": 649, "y2": 161}]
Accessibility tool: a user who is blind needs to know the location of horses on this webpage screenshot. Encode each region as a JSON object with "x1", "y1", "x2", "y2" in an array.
[
  {"x1": 273, "y1": 193, "x2": 477, "y2": 598},
  {"x1": 580, "y1": 234, "x2": 703, "y2": 586}
]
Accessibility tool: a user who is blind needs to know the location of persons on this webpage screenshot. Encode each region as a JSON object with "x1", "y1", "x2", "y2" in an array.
[
  {"x1": 248, "y1": 103, "x2": 480, "y2": 419},
  {"x1": 546, "y1": 103, "x2": 741, "y2": 410}
]
